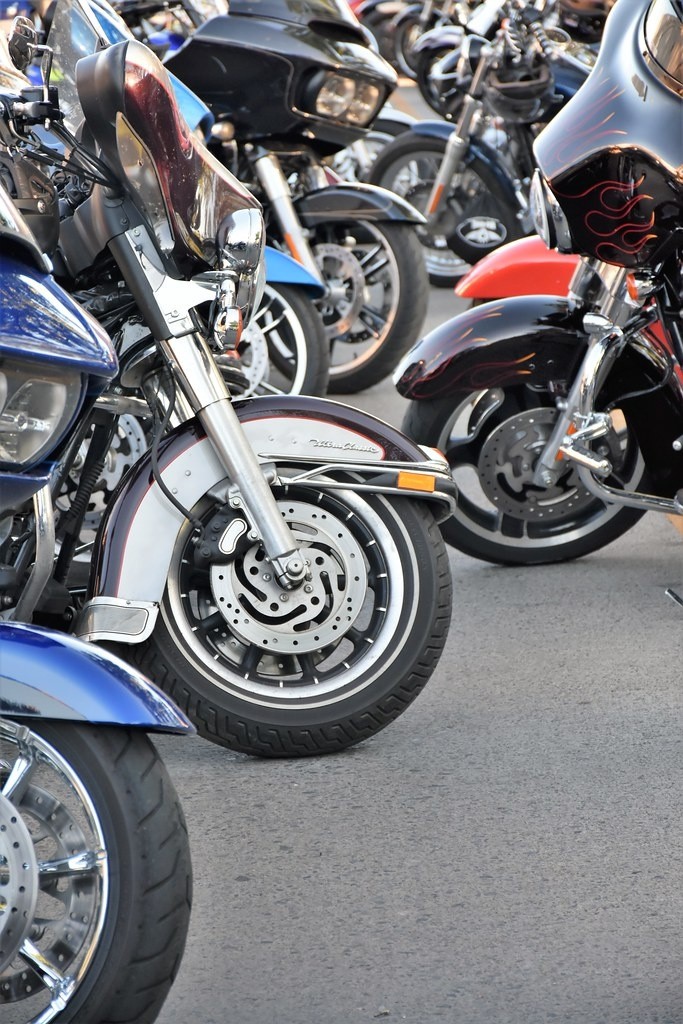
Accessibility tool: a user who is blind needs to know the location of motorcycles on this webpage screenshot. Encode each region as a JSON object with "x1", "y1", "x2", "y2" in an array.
[{"x1": 0, "y1": 0, "x2": 683, "y2": 1024}]
